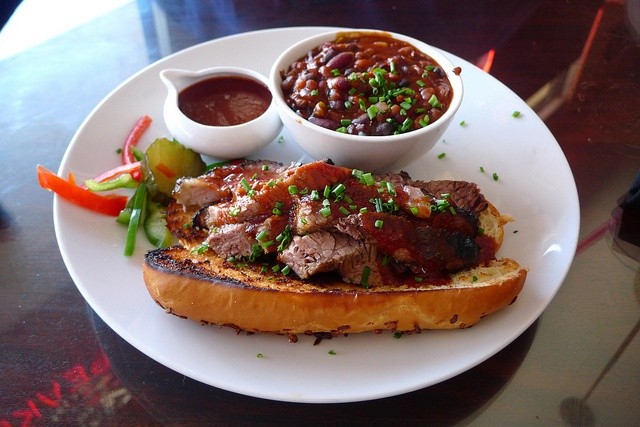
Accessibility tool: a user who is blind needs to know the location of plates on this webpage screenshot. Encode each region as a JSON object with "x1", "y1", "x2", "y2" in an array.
[{"x1": 52, "y1": 26, "x2": 582, "y2": 408}]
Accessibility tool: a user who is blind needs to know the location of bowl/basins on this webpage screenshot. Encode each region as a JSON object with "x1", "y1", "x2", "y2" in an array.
[
  {"x1": 270, "y1": 28, "x2": 463, "y2": 174},
  {"x1": 159, "y1": 63, "x2": 282, "y2": 159}
]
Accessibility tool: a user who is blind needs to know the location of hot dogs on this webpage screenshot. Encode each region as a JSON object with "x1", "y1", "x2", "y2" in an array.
[{"x1": 142, "y1": 160, "x2": 530, "y2": 344}]
